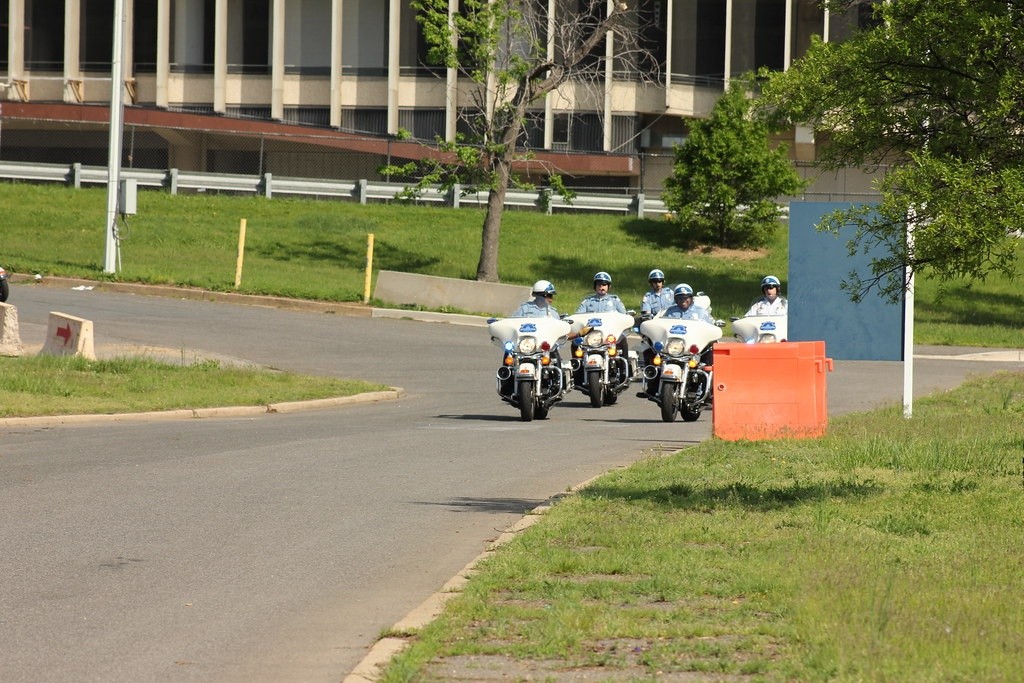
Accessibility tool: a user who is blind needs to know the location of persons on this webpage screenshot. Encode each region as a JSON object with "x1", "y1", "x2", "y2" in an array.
[
  {"x1": 500, "y1": 279, "x2": 594, "y2": 402},
  {"x1": 636, "y1": 268, "x2": 712, "y2": 398},
  {"x1": 744, "y1": 275, "x2": 788, "y2": 317},
  {"x1": 571, "y1": 272, "x2": 628, "y2": 390}
]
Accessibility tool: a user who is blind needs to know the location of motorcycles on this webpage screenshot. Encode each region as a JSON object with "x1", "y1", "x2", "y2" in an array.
[
  {"x1": 559, "y1": 311, "x2": 792, "y2": 423},
  {"x1": 486, "y1": 316, "x2": 573, "y2": 422}
]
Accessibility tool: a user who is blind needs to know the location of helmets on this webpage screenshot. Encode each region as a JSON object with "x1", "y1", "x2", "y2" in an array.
[
  {"x1": 532, "y1": 280, "x2": 557, "y2": 295},
  {"x1": 761, "y1": 276, "x2": 781, "y2": 295},
  {"x1": 648, "y1": 269, "x2": 665, "y2": 285},
  {"x1": 674, "y1": 283, "x2": 693, "y2": 305},
  {"x1": 594, "y1": 272, "x2": 612, "y2": 290}
]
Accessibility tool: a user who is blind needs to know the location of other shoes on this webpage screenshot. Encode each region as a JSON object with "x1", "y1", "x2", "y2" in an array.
[{"x1": 636, "y1": 390, "x2": 658, "y2": 398}]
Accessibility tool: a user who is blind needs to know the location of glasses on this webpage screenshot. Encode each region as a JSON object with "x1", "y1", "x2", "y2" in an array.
[
  {"x1": 675, "y1": 295, "x2": 690, "y2": 300},
  {"x1": 652, "y1": 278, "x2": 662, "y2": 283},
  {"x1": 764, "y1": 286, "x2": 774, "y2": 289},
  {"x1": 596, "y1": 281, "x2": 608, "y2": 285},
  {"x1": 545, "y1": 294, "x2": 553, "y2": 298}
]
